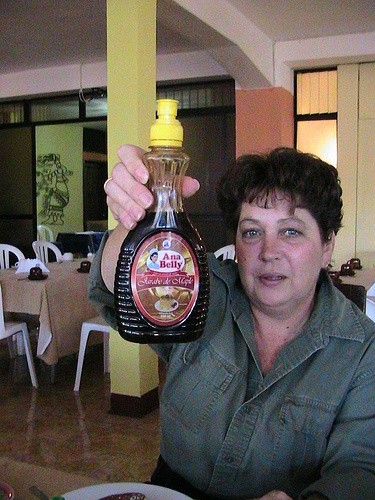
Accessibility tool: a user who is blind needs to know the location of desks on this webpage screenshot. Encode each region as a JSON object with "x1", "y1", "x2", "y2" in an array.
[
  {"x1": 328, "y1": 266, "x2": 375, "y2": 291},
  {"x1": 56, "y1": 232, "x2": 104, "y2": 258},
  {"x1": 0, "y1": 258, "x2": 106, "y2": 383}
]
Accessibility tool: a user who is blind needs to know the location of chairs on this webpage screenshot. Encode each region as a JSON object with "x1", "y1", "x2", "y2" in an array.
[
  {"x1": 0, "y1": 285, "x2": 39, "y2": 389},
  {"x1": 37, "y1": 224, "x2": 54, "y2": 243},
  {"x1": 73, "y1": 315, "x2": 110, "y2": 391},
  {"x1": 32, "y1": 241, "x2": 62, "y2": 263},
  {"x1": 0, "y1": 244, "x2": 25, "y2": 270}
]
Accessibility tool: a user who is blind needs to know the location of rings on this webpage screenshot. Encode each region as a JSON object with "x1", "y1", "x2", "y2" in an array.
[{"x1": 104, "y1": 178, "x2": 112, "y2": 188}]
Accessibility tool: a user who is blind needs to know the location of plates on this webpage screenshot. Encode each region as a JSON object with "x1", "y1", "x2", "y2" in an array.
[
  {"x1": 76, "y1": 268, "x2": 90, "y2": 273},
  {"x1": 332, "y1": 279, "x2": 343, "y2": 284},
  {"x1": 27, "y1": 275, "x2": 48, "y2": 280},
  {"x1": 53, "y1": 481, "x2": 193, "y2": 500},
  {"x1": 353, "y1": 266, "x2": 363, "y2": 270},
  {"x1": 339, "y1": 273, "x2": 356, "y2": 276}
]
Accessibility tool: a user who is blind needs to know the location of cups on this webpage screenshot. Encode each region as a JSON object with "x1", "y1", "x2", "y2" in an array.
[
  {"x1": 80, "y1": 261, "x2": 91, "y2": 270},
  {"x1": 30, "y1": 268, "x2": 42, "y2": 277},
  {"x1": 341, "y1": 264, "x2": 353, "y2": 273},
  {"x1": 60, "y1": 252, "x2": 74, "y2": 261},
  {"x1": 0, "y1": 481, "x2": 14, "y2": 500},
  {"x1": 327, "y1": 272, "x2": 339, "y2": 283},
  {"x1": 346, "y1": 258, "x2": 360, "y2": 267},
  {"x1": 14, "y1": 259, "x2": 27, "y2": 268}
]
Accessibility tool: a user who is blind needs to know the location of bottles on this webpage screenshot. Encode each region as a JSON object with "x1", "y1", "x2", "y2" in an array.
[{"x1": 113, "y1": 99, "x2": 209, "y2": 343}]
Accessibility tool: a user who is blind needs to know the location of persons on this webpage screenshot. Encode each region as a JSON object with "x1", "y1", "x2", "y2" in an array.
[
  {"x1": 148, "y1": 249, "x2": 160, "y2": 270},
  {"x1": 87, "y1": 143, "x2": 375, "y2": 500}
]
